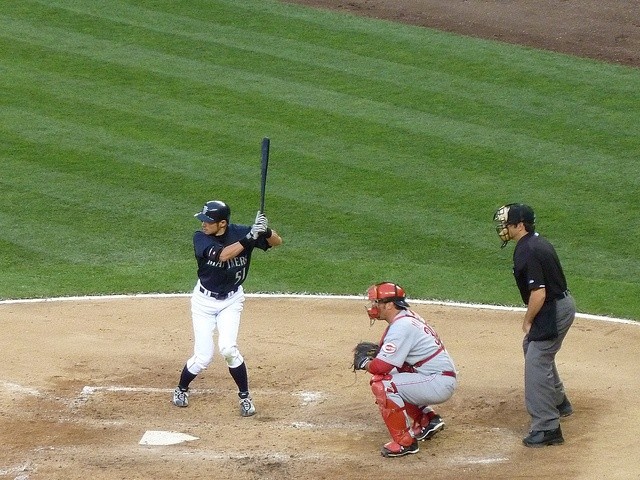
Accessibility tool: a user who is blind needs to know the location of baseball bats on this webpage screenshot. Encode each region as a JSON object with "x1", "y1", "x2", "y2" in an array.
[{"x1": 259, "y1": 137, "x2": 270, "y2": 235}]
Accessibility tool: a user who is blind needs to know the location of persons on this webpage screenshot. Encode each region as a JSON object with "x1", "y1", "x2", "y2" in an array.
[
  {"x1": 492, "y1": 203, "x2": 577, "y2": 447},
  {"x1": 352, "y1": 282, "x2": 457, "y2": 458},
  {"x1": 173, "y1": 199, "x2": 282, "y2": 418}
]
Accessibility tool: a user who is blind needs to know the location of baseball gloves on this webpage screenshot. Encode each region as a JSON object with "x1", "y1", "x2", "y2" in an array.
[{"x1": 353, "y1": 342, "x2": 380, "y2": 370}]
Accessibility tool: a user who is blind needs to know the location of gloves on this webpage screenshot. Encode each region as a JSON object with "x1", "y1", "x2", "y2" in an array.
[
  {"x1": 252, "y1": 223, "x2": 272, "y2": 239},
  {"x1": 239, "y1": 210, "x2": 266, "y2": 248}
]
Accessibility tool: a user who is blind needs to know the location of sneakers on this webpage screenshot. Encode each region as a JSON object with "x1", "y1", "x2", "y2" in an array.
[
  {"x1": 416, "y1": 414, "x2": 445, "y2": 442},
  {"x1": 173, "y1": 386, "x2": 190, "y2": 407},
  {"x1": 238, "y1": 397, "x2": 256, "y2": 417},
  {"x1": 557, "y1": 403, "x2": 574, "y2": 417},
  {"x1": 380, "y1": 437, "x2": 419, "y2": 456},
  {"x1": 522, "y1": 425, "x2": 564, "y2": 448}
]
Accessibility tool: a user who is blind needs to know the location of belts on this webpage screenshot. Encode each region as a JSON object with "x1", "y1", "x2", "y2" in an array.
[
  {"x1": 556, "y1": 291, "x2": 569, "y2": 301},
  {"x1": 198, "y1": 286, "x2": 238, "y2": 300},
  {"x1": 443, "y1": 371, "x2": 456, "y2": 377}
]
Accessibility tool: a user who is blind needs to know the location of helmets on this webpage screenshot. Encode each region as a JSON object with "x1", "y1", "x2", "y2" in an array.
[
  {"x1": 364, "y1": 281, "x2": 410, "y2": 327},
  {"x1": 194, "y1": 200, "x2": 231, "y2": 225},
  {"x1": 492, "y1": 202, "x2": 535, "y2": 249}
]
